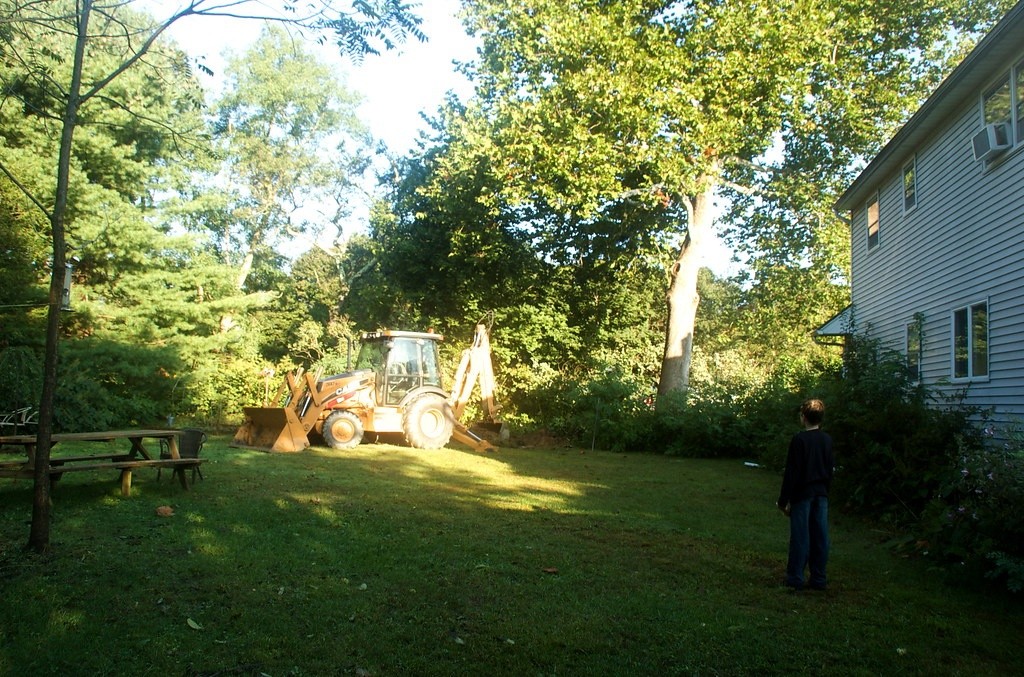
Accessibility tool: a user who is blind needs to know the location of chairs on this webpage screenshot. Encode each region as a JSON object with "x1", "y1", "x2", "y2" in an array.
[{"x1": 157, "y1": 429, "x2": 207, "y2": 484}]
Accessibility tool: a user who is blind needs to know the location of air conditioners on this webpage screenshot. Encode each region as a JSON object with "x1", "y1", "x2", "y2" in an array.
[{"x1": 971, "y1": 122, "x2": 1013, "y2": 162}]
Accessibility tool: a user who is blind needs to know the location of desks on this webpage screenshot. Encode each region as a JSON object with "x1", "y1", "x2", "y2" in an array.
[{"x1": 0, "y1": 429, "x2": 189, "y2": 507}]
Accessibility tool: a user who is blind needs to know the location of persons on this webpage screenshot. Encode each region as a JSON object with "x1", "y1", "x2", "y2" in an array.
[{"x1": 777, "y1": 399, "x2": 836, "y2": 596}]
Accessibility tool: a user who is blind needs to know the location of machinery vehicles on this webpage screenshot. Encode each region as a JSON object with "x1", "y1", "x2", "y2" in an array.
[{"x1": 229, "y1": 323, "x2": 512, "y2": 455}]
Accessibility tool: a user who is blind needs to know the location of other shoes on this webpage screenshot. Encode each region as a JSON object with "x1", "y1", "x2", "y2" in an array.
[{"x1": 803, "y1": 581, "x2": 829, "y2": 593}]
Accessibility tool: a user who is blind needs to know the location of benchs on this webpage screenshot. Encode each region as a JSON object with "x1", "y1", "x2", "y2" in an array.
[{"x1": 0, "y1": 453, "x2": 210, "y2": 506}]
